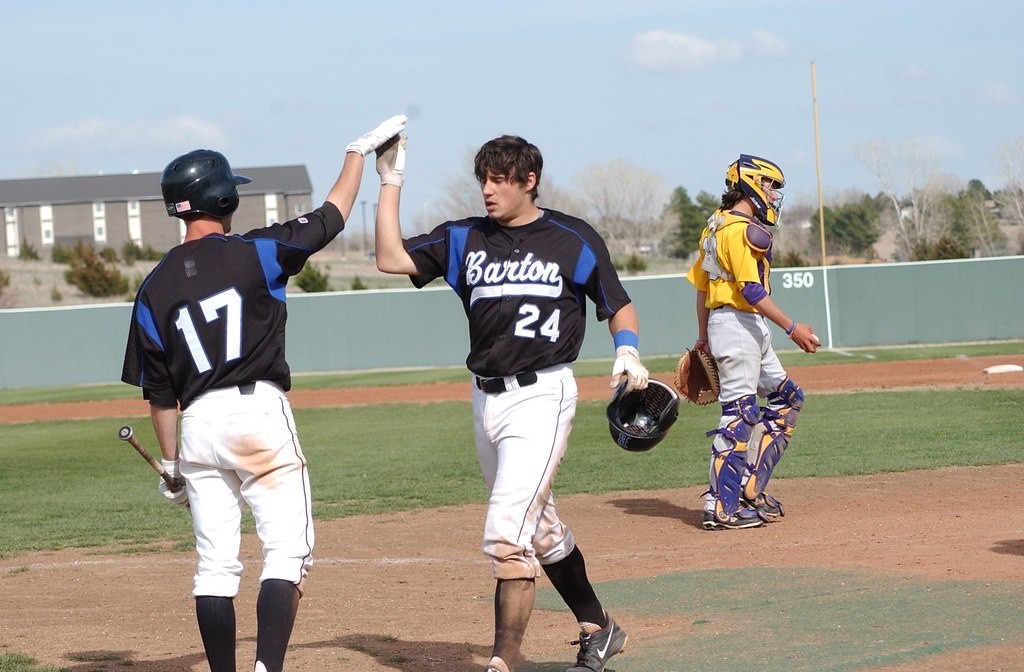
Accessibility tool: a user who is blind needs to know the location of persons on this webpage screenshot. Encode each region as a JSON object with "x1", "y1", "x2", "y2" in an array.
[
  {"x1": 374, "y1": 123, "x2": 648, "y2": 671},
  {"x1": 119, "y1": 112, "x2": 407, "y2": 667},
  {"x1": 684, "y1": 154, "x2": 822, "y2": 532}
]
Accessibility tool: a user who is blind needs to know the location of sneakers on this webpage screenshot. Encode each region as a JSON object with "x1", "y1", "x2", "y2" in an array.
[
  {"x1": 740, "y1": 490, "x2": 780, "y2": 517},
  {"x1": 565, "y1": 611, "x2": 627, "y2": 672},
  {"x1": 702, "y1": 508, "x2": 763, "y2": 531}
]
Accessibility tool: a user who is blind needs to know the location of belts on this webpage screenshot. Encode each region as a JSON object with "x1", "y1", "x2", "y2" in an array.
[
  {"x1": 713, "y1": 305, "x2": 764, "y2": 319},
  {"x1": 476, "y1": 371, "x2": 538, "y2": 394},
  {"x1": 238, "y1": 380, "x2": 256, "y2": 396}
]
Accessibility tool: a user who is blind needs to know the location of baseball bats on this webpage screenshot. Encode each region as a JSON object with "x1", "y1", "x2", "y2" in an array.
[{"x1": 117, "y1": 427, "x2": 190, "y2": 512}]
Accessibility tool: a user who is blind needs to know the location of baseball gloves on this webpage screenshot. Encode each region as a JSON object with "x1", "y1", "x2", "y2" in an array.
[{"x1": 676, "y1": 348, "x2": 721, "y2": 404}]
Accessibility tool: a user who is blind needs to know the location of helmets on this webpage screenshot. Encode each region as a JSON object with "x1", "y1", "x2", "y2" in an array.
[
  {"x1": 725, "y1": 153, "x2": 784, "y2": 226},
  {"x1": 160, "y1": 149, "x2": 252, "y2": 219},
  {"x1": 607, "y1": 376, "x2": 680, "y2": 452}
]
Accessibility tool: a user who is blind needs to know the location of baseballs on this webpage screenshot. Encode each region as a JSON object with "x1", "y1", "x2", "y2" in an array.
[{"x1": 804, "y1": 332, "x2": 818, "y2": 349}]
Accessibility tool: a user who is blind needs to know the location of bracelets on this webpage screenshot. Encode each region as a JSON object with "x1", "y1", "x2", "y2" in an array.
[{"x1": 786, "y1": 320, "x2": 797, "y2": 341}]
